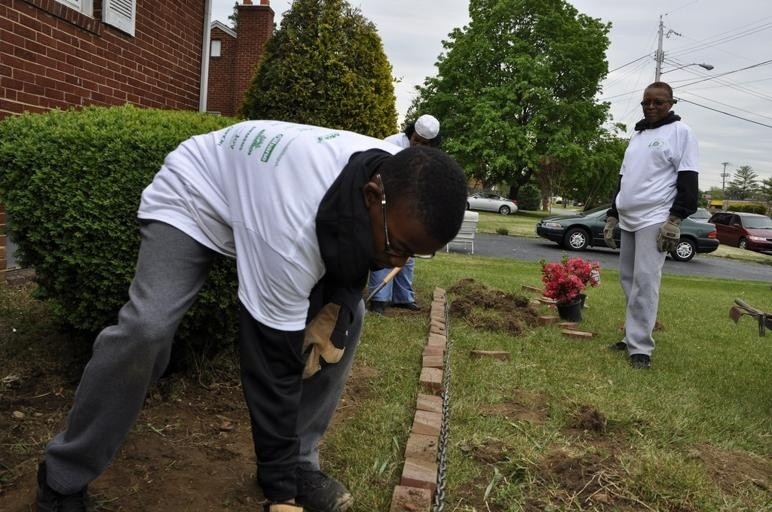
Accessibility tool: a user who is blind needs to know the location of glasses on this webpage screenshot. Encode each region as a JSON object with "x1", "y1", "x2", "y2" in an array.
[
  {"x1": 639, "y1": 98, "x2": 673, "y2": 105},
  {"x1": 376, "y1": 171, "x2": 439, "y2": 261}
]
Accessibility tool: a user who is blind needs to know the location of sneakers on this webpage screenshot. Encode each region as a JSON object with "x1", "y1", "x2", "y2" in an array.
[
  {"x1": 368, "y1": 298, "x2": 385, "y2": 314},
  {"x1": 612, "y1": 341, "x2": 651, "y2": 369},
  {"x1": 391, "y1": 300, "x2": 420, "y2": 311},
  {"x1": 252, "y1": 462, "x2": 356, "y2": 511}
]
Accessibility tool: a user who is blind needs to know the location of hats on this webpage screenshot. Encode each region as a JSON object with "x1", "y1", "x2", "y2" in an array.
[{"x1": 414, "y1": 113, "x2": 440, "y2": 140}]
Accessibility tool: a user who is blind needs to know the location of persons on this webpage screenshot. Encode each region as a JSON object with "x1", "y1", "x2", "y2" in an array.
[
  {"x1": 35, "y1": 116, "x2": 469, "y2": 511},
  {"x1": 366, "y1": 112, "x2": 445, "y2": 315},
  {"x1": 602, "y1": 80, "x2": 700, "y2": 371}
]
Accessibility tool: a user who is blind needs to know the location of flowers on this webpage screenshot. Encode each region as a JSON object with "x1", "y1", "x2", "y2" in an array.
[
  {"x1": 537, "y1": 259, "x2": 588, "y2": 304},
  {"x1": 560, "y1": 251, "x2": 601, "y2": 292}
]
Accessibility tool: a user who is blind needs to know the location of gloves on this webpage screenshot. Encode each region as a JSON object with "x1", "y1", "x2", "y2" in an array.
[
  {"x1": 299, "y1": 295, "x2": 352, "y2": 381},
  {"x1": 655, "y1": 220, "x2": 680, "y2": 252},
  {"x1": 603, "y1": 219, "x2": 620, "y2": 248}
]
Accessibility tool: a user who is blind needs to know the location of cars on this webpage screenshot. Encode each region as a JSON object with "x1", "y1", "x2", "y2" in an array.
[
  {"x1": 537, "y1": 199, "x2": 772, "y2": 262},
  {"x1": 465, "y1": 192, "x2": 519, "y2": 215}
]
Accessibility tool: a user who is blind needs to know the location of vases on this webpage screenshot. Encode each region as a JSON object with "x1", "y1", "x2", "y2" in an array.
[
  {"x1": 558, "y1": 296, "x2": 581, "y2": 322},
  {"x1": 579, "y1": 295, "x2": 586, "y2": 307}
]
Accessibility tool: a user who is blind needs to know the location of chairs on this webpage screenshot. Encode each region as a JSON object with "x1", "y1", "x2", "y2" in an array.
[{"x1": 442, "y1": 209, "x2": 480, "y2": 254}]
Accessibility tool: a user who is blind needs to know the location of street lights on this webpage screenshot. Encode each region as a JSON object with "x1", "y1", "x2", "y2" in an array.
[{"x1": 656, "y1": 14, "x2": 714, "y2": 83}]
